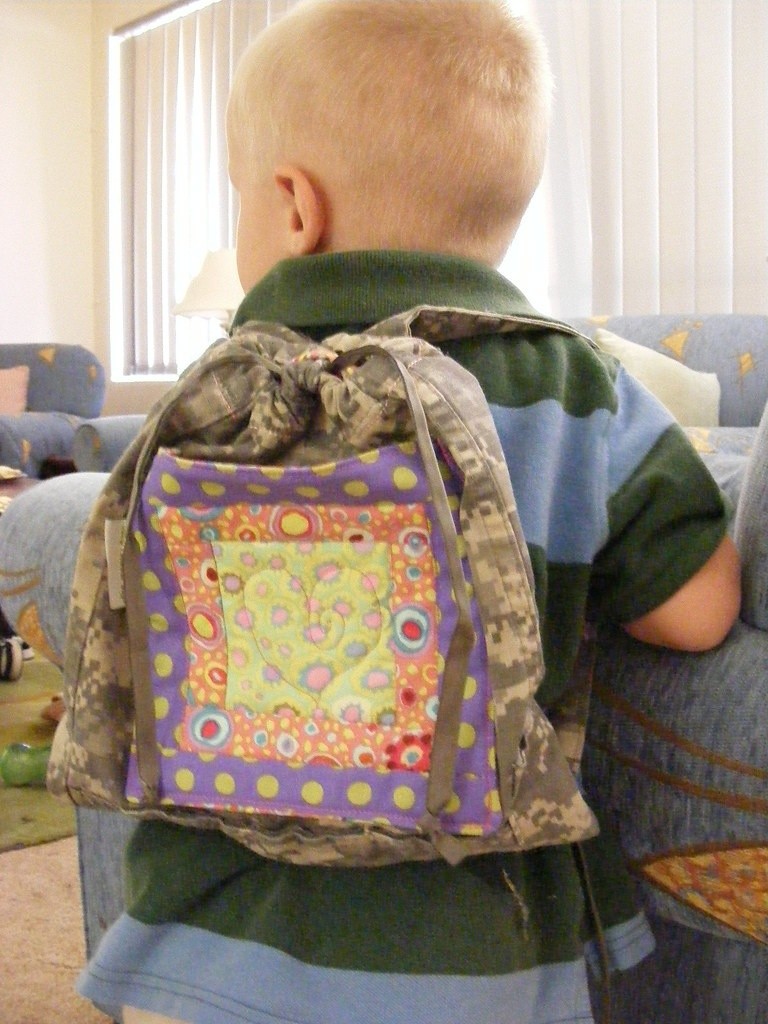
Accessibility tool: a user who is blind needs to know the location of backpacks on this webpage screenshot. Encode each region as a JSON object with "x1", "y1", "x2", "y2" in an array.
[{"x1": 44, "y1": 302, "x2": 603, "y2": 869}]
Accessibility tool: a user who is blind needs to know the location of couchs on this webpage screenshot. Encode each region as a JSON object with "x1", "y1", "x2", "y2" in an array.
[
  {"x1": 71, "y1": 312, "x2": 768, "y2": 535},
  {"x1": 0, "y1": 342, "x2": 105, "y2": 480},
  {"x1": 0, "y1": 407, "x2": 768, "y2": 1024}
]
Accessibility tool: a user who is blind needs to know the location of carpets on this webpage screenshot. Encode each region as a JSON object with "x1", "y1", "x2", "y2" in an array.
[{"x1": 0, "y1": 660, "x2": 78, "y2": 852}]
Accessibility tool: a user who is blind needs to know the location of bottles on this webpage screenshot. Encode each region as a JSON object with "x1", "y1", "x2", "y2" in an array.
[{"x1": 0, "y1": 745, "x2": 51, "y2": 785}]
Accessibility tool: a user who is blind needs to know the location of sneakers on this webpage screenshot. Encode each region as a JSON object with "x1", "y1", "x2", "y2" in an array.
[{"x1": 0, "y1": 636, "x2": 35, "y2": 682}]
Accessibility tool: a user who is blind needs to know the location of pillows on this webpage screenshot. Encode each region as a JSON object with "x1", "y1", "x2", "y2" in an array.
[
  {"x1": 597, "y1": 328, "x2": 722, "y2": 427},
  {"x1": 0, "y1": 365, "x2": 30, "y2": 413}
]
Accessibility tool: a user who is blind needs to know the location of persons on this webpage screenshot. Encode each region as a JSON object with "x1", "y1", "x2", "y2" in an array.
[{"x1": 78, "y1": 0, "x2": 741, "y2": 1024}]
